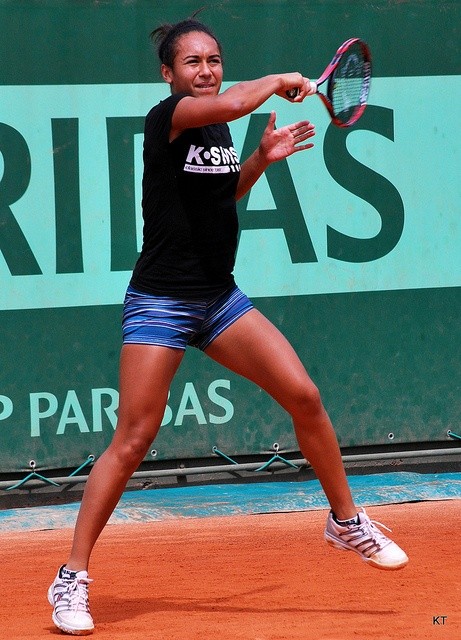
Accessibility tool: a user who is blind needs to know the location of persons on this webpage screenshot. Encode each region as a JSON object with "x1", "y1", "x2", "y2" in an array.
[{"x1": 46, "y1": 20, "x2": 408, "y2": 636}]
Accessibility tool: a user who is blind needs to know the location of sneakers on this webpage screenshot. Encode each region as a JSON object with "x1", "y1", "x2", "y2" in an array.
[
  {"x1": 325, "y1": 505, "x2": 409, "y2": 571},
  {"x1": 47, "y1": 564, "x2": 94, "y2": 635}
]
